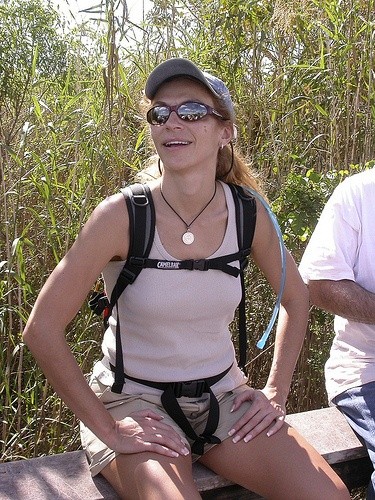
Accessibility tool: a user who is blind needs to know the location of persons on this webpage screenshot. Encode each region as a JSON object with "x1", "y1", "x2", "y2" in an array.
[
  {"x1": 21, "y1": 58, "x2": 351, "y2": 500},
  {"x1": 297, "y1": 163, "x2": 375, "y2": 500}
]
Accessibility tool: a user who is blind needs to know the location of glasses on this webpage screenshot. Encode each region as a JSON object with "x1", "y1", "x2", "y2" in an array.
[{"x1": 146, "y1": 101, "x2": 230, "y2": 125}]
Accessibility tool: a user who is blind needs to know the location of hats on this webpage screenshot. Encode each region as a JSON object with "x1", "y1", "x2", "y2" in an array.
[{"x1": 144, "y1": 58, "x2": 235, "y2": 121}]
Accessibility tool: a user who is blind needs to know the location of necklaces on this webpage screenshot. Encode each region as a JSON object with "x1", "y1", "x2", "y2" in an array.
[{"x1": 160, "y1": 179, "x2": 217, "y2": 246}]
